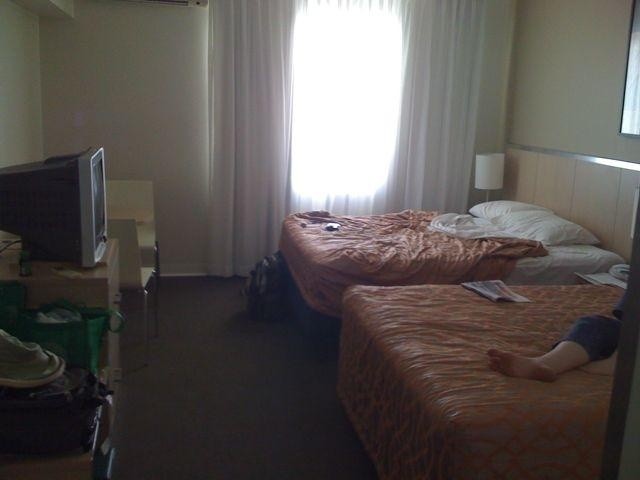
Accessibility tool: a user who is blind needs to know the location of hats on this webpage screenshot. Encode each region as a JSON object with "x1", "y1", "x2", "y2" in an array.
[{"x1": 0, "y1": 327, "x2": 67, "y2": 389}]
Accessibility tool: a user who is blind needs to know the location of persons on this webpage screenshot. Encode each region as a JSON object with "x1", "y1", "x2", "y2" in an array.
[{"x1": 487, "y1": 314, "x2": 623, "y2": 380}]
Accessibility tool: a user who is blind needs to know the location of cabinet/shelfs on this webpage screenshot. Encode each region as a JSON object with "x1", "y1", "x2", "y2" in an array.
[{"x1": 0, "y1": 239, "x2": 123, "y2": 477}]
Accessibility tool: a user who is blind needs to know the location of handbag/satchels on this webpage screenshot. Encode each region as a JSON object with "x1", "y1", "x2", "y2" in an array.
[{"x1": 12, "y1": 298, "x2": 127, "y2": 382}]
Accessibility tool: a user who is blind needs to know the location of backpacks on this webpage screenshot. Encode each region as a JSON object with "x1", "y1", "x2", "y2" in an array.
[{"x1": 246, "y1": 250, "x2": 290, "y2": 324}]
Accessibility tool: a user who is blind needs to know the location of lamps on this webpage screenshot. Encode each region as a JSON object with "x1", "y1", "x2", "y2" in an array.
[{"x1": 474, "y1": 151, "x2": 505, "y2": 203}]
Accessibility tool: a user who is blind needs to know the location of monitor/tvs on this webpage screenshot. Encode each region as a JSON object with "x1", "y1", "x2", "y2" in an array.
[{"x1": 0, "y1": 143, "x2": 108, "y2": 268}]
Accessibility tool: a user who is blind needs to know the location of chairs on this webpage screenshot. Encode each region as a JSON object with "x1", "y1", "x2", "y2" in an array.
[{"x1": 103, "y1": 177, "x2": 162, "y2": 369}]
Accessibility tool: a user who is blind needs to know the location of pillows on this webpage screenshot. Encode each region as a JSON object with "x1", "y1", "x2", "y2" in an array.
[
  {"x1": 468, "y1": 200, "x2": 556, "y2": 218},
  {"x1": 490, "y1": 210, "x2": 600, "y2": 247}
]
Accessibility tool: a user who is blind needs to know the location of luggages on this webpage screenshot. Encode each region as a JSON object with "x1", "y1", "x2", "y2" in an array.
[{"x1": 0, "y1": 363, "x2": 115, "y2": 455}]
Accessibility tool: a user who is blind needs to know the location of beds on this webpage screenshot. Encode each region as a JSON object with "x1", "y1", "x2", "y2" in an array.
[
  {"x1": 335, "y1": 282, "x2": 637, "y2": 477},
  {"x1": 277, "y1": 146, "x2": 637, "y2": 335}
]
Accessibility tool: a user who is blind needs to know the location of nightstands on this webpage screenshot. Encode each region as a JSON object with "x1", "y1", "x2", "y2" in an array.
[{"x1": 574, "y1": 270, "x2": 626, "y2": 285}]
